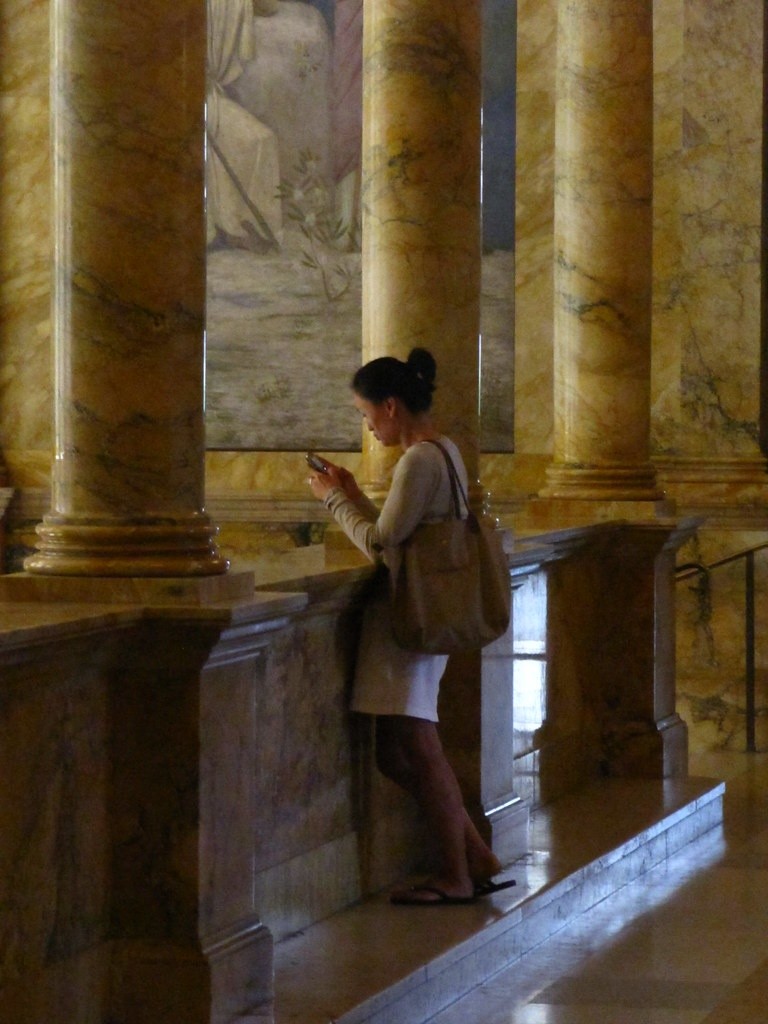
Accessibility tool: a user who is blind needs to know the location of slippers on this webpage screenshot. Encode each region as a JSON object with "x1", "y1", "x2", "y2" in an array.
[
  {"x1": 474, "y1": 877, "x2": 516, "y2": 897},
  {"x1": 391, "y1": 885, "x2": 478, "y2": 904}
]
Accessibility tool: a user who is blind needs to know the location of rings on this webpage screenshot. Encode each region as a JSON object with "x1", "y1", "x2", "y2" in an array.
[{"x1": 310, "y1": 478, "x2": 312, "y2": 484}]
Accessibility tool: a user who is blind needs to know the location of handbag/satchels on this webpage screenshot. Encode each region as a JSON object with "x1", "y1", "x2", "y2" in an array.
[{"x1": 393, "y1": 439, "x2": 511, "y2": 655}]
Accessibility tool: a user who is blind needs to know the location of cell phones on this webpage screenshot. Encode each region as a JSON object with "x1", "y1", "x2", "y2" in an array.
[{"x1": 305, "y1": 451, "x2": 326, "y2": 473}]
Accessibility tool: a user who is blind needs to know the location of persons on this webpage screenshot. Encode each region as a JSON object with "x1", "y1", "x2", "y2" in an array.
[{"x1": 306, "y1": 347, "x2": 502, "y2": 904}]
